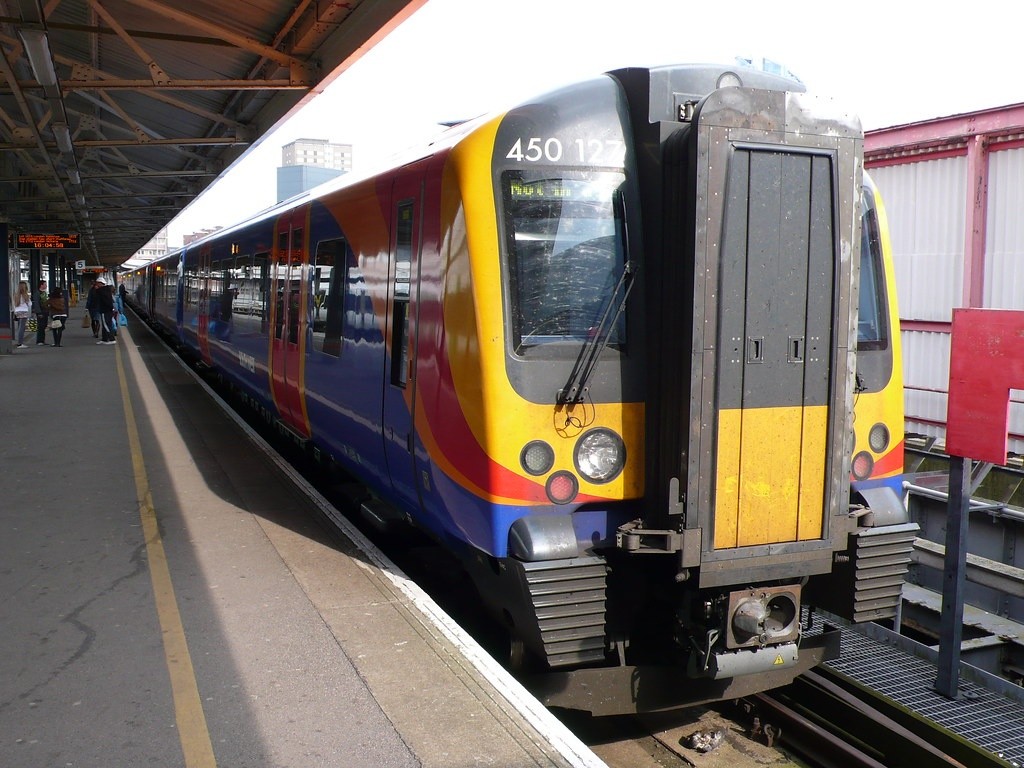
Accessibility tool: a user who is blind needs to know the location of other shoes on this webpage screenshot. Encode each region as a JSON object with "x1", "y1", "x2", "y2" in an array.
[
  {"x1": 96, "y1": 341, "x2": 104, "y2": 344},
  {"x1": 17, "y1": 344, "x2": 29, "y2": 348},
  {"x1": 48, "y1": 343, "x2": 55, "y2": 346},
  {"x1": 37, "y1": 343, "x2": 44, "y2": 346},
  {"x1": 95, "y1": 334, "x2": 98, "y2": 338},
  {"x1": 103, "y1": 341, "x2": 116, "y2": 345}
]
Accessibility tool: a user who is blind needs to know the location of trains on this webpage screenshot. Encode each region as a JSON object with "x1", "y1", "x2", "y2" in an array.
[{"x1": 121, "y1": 57, "x2": 920, "y2": 716}]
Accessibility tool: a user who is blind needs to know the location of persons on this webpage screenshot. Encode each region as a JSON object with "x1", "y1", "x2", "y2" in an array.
[
  {"x1": 43, "y1": 287, "x2": 66, "y2": 347},
  {"x1": 96, "y1": 277, "x2": 117, "y2": 345},
  {"x1": 33, "y1": 280, "x2": 50, "y2": 346},
  {"x1": 119, "y1": 281, "x2": 129, "y2": 307},
  {"x1": 14, "y1": 281, "x2": 32, "y2": 348},
  {"x1": 314, "y1": 291, "x2": 322, "y2": 319},
  {"x1": 85, "y1": 284, "x2": 123, "y2": 338}
]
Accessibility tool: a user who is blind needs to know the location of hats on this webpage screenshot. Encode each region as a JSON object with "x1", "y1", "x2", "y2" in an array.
[{"x1": 96, "y1": 277, "x2": 107, "y2": 285}]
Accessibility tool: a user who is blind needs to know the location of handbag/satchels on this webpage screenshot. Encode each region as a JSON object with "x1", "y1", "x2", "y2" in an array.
[
  {"x1": 81, "y1": 311, "x2": 90, "y2": 328},
  {"x1": 119, "y1": 313, "x2": 127, "y2": 326},
  {"x1": 52, "y1": 320, "x2": 62, "y2": 329}
]
